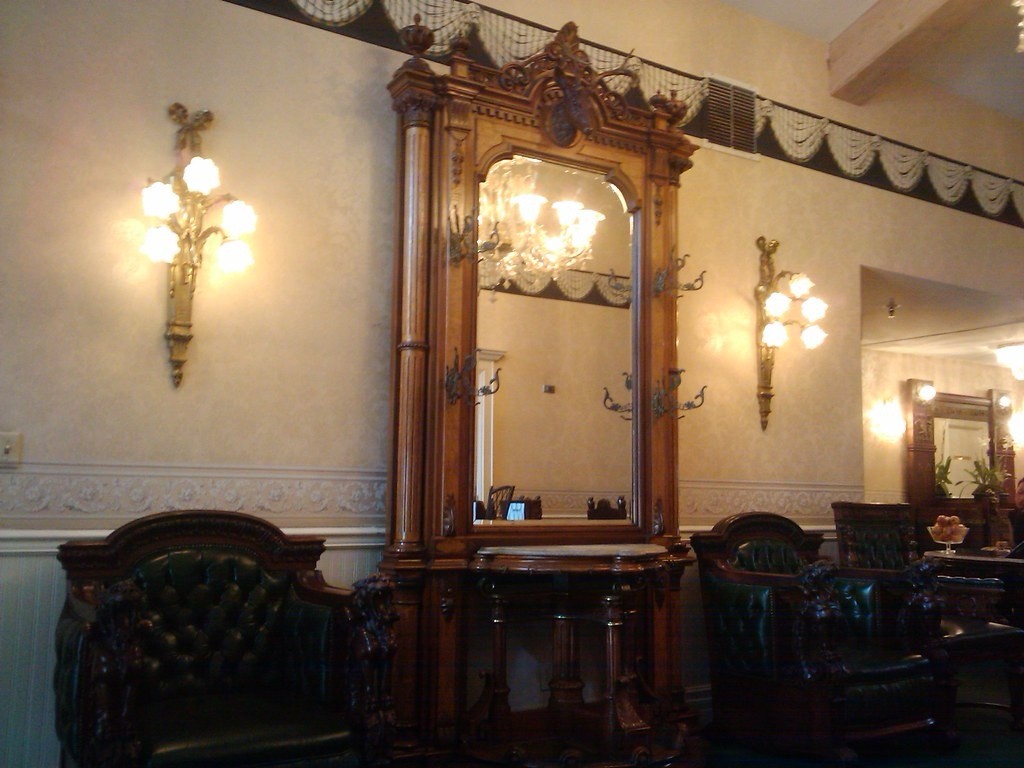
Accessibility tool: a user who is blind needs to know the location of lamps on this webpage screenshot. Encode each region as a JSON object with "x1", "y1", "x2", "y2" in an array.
[
  {"x1": 138, "y1": 101, "x2": 259, "y2": 391},
  {"x1": 918, "y1": 382, "x2": 937, "y2": 402},
  {"x1": 478, "y1": 160, "x2": 606, "y2": 276},
  {"x1": 755, "y1": 235, "x2": 830, "y2": 433}
]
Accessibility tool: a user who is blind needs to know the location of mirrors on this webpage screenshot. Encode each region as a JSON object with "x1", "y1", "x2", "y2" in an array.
[
  {"x1": 385, "y1": 11, "x2": 710, "y2": 550},
  {"x1": 927, "y1": 413, "x2": 1001, "y2": 499}
]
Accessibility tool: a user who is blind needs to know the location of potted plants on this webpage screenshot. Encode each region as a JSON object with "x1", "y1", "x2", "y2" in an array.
[
  {"x1": 931, "y1": 453, "x2": 975, "y2": 499},
  {"x1": 953, "y1": 457, "x2": 1018, "y2": 506}
]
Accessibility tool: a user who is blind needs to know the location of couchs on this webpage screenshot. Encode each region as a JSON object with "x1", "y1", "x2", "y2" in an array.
[
  {"x1": 50, "y1": 508, "x2": 403, "y2": 768},
  {"x1": 688, "y1": 510, "x2": 963, "y2": 768}
]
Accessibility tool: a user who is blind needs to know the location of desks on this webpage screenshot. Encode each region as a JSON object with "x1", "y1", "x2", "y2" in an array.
[
  {"x1": 475, "y1": 542, "x2": 671, "y2": 768},
  {"x1": 923, "y1": 549, "x2": 1023, "y2": 630}
]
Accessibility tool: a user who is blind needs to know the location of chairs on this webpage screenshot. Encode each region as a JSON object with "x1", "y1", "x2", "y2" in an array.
[
  {"x1": 832, "y1": 501, "x2": 1024, "y2": 724},
  {"x1": 482, "y1": 483, "x2": 517, "y2": 521},
  {"x1": 586, "y1": 492, "x2": 629, "y2": 520}
]
